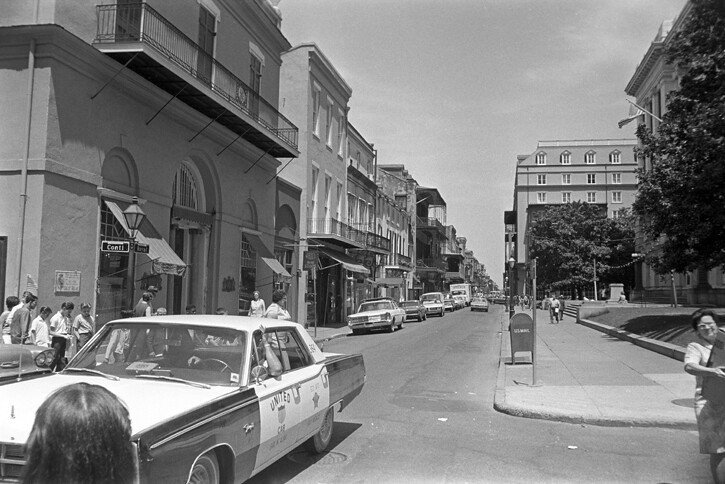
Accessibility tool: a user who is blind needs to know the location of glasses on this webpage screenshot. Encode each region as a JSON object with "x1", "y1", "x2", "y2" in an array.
[{"x1": 697, "y1": 321, "x2": 716, "y2": 331}]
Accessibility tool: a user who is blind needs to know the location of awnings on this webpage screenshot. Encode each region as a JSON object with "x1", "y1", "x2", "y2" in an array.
[
  {"x1": 98, "y1": 197, "x2": 187, "y2": 283},
  {"x1": 316, "y1": 246, "x2": 371, "y2": 275},
  {"x1": 243, "y1": 232, "x2": 292, "y2": 289}
]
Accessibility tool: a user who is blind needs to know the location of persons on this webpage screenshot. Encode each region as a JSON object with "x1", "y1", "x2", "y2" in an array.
[
  {"x1": 548, "y1": 294, "x2": 565, "y2": 324},
  {"x1": 0, "y1": 285, "x2": 293, "y2": 375},
  {"x1": 683, "y1": 307, "x2": 725, "y2": 484},
  {"x1": 17, "y1": 381, "x2": 138, "y2": 484}
]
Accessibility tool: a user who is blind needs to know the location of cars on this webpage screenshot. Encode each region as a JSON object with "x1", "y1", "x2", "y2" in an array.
[
  {"x1": 418, "y1": 283, "x2": 519, "y2": 316},
  {"x1": 0, "y1": 329, "x2": 62, "y2": 387},
  {"x1": 397, "y1": 300, "x2": 429, "y2": 323},
  {"x1": 348, "y1": 297, "x2": 406, "y2": 333},
  {"x1": 0, "y1": 313, "x2": 366, "y2": 484}
]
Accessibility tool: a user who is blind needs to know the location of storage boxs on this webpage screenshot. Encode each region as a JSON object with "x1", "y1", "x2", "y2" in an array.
[{"x1": 701, "y1": 327, "x2": 725, "y2": 408}]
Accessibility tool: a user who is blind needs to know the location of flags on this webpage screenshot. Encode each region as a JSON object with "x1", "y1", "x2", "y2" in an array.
[{"x1": 617, "y1": 102, "x2": 646, "y2": 129}]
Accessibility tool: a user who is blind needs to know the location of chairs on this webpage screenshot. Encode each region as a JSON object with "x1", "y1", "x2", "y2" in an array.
[{"x1": 190, "y1": 350, "x2": 302, "y2": 379}]
